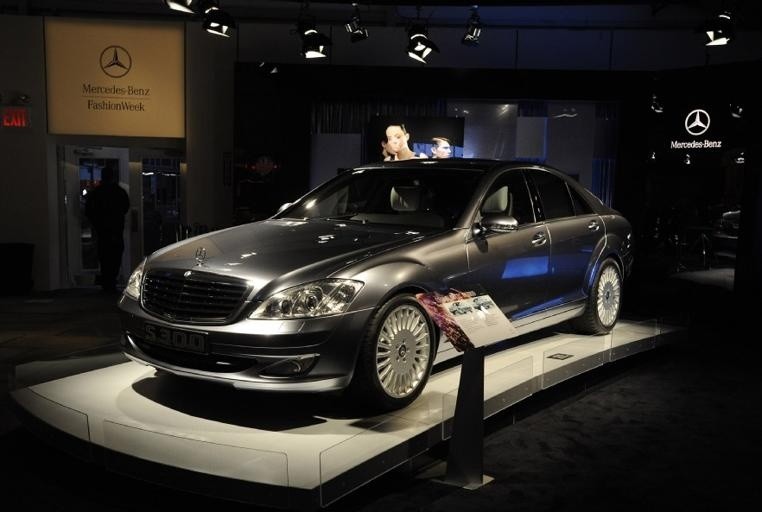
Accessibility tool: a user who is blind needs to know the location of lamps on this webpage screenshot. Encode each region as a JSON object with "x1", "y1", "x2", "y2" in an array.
[
  {"x1": 163, "y1": 0, "x2": 233, "y2": 40},
  {"x1": 702, "y1": 2, "x2": 735, "y2": 47},
  {"x1": 286, "y1": 3, "x2": 334, "y2": 62},
  {"x1": 397, "y1": 7, "x2": 441, "y2": 66},
  {"x1": 342, "y1": 2, "x2": 371, "y2": 44},
  {"x1": 460, "y1": 5, "x2": 485, "y2": 50}
]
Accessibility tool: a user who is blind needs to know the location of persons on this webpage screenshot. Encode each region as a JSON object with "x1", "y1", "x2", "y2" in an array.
[
  {"x1": 430, "y1": 136, "x2": 451, "y2": 160},
  {"x1": 85, "y1": 167, "x2": 130, "y2": 294},
  {"x1": 382, "y1": 123, "x2": 428, "y2": 162}
]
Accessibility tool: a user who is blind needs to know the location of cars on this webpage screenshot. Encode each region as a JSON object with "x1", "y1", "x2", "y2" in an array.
[
  {"x1": 669, "y1": 195, "x2": 740, "y2": 267},
  {"x1": 118, "y1": 157, "x2": 634, "y2": 410}
]
